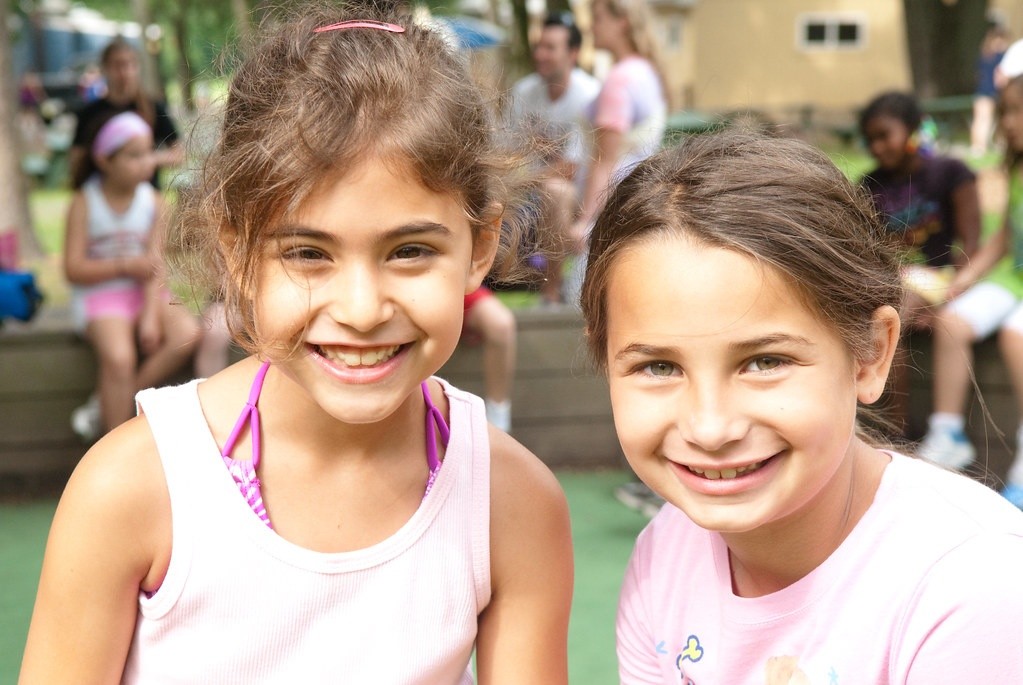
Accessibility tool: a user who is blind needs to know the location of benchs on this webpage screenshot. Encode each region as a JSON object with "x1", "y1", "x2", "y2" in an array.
[{"x1": 1, "y1": 302, "x2": 1022, "y2": 500}]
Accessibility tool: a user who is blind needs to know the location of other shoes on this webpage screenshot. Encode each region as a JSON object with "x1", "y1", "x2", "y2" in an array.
[
  {"x1": 910, "y1": 430, "x2": 978, "y2": 472},
  {"x1": 69, "y1": 392, "x2": 103, "y2": 442}
]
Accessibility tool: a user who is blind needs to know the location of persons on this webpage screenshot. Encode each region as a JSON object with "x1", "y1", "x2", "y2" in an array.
[
  {"x1": 19, "y1": 6, "x2": 574, "y2": 685},
  {"x1": 583, "y1": 131, "x2": 1023, "y2": 685},
  {"x1": 17, "y1": 36, "x2": 244, "y2": 443},
  {"x1": 849, "y1": 9, "x2": 1023, "y2": 508},
  {"x1": 456, "y1": 0, "x2": 669, "y2": 449}
]
